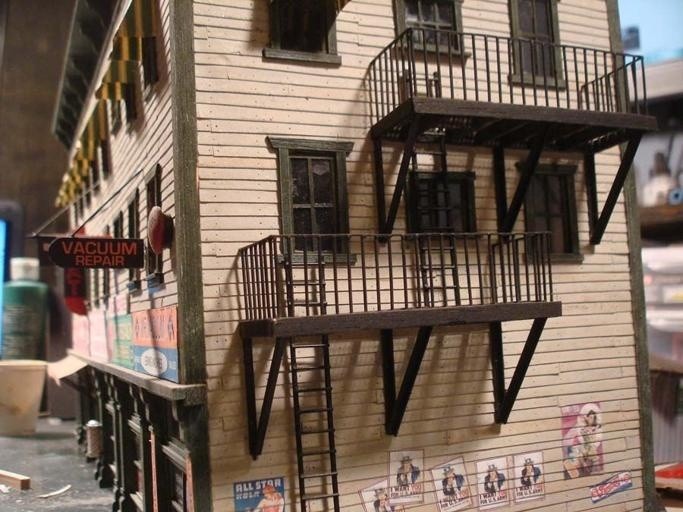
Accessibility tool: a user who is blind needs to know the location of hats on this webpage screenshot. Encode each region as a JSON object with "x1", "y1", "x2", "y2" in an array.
[
  {"x1": 442, "y1": 466, "x2": 454, "y2": 475},
  {"x1": 400, "y1": 455, "x2": 412, "y2": 463},
  {"x1": 523, "y1": 458, "x2": 533, "y2": 466},
  {"x1": 262, "y1": 486, "x2": 275, "y2": 494},
  {"x1": 373, "y1": 488, "x2": 387, "y2": 497},
  {"x1": 487, "y1": 465, "x2": 497, "y2": 472}
]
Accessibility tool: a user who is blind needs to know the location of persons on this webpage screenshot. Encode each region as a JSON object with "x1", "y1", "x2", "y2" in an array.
[
  {"x1": 568, "y1": 410, "x2": 603, "y2": 477},
  {"x1": 253, "y1": 484, "x2": 284, "y2": 512},
  {"x1": 398, "y1": 455, "x2": 421, "y2": 494},
  {"x1": 521, "y1": 457, "x2": 541, "y2": 496},
  {"x1": 484, "y1": 464, "x2": 505, "y2": 500},
  {"x1": 373, "y1": 489, "x2": 396, "y2": 512},
  {"x1": 441, "y1": 466, "x2": 468, "y2": 501}
]
